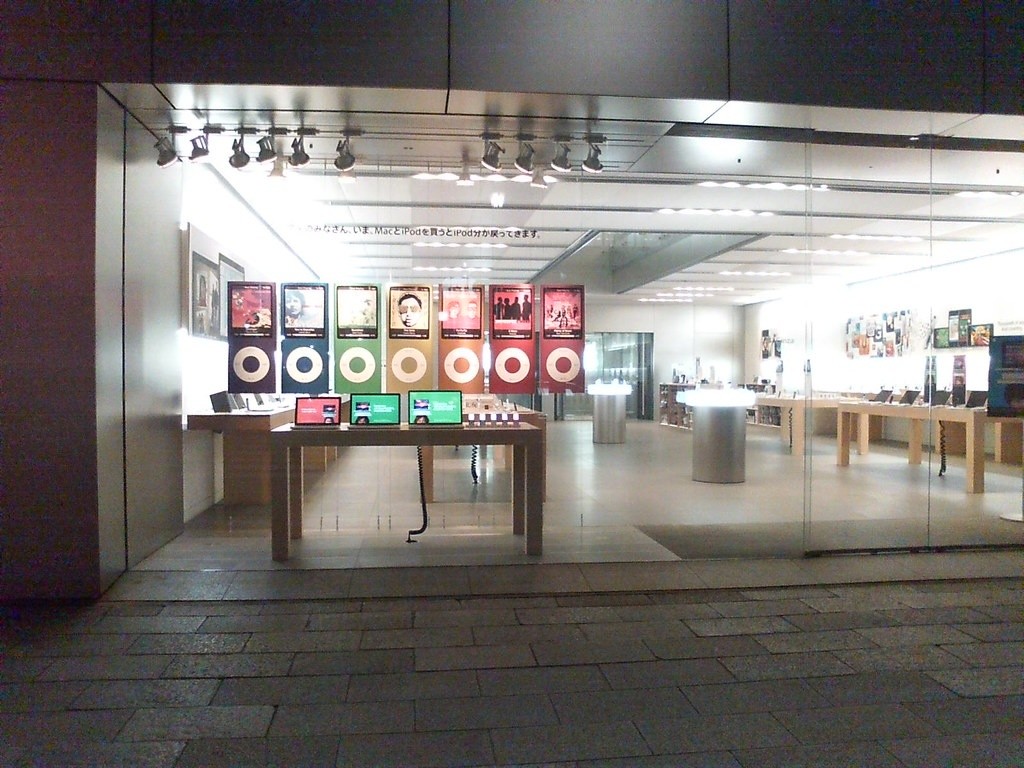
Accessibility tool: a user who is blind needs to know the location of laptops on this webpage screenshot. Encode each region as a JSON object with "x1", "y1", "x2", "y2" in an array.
[
  {"x1": 858, "y1": 390, "x2": 988, "y2": 410},
  {"x1": 347, "y1": 393, "x2": 401, "y2": 429},
  {"x1": 292, "y1": 397, "x2": 341, "y2": 429},
  {"x1": 408, "y1": 390, "x2": 464, "y2": 429}
]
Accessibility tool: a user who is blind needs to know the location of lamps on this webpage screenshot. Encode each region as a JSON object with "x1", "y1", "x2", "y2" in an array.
[
  {"x1": 227, "y1": 128, "x2": 261, "y2": 170},
  {"x1": 477, "y1": 132, "x2": 506, "y2": 173},
  {"x1": 155, "y1": 127, "x2": 192, "y2": 169},
  {"x1": 551, "y1": 136, "x2": 574, "y2": 173},
  {"x1": 256, "y1": 128, "x2": 290, "y2": 165},
  {"x1": 515, "y1": 134, "x2": 538, "y2": 175},
  {"x1": 334, "y1": 131, "x2": 364, "y2": 172},
  {"x1": 188, "y1": 127, "x2": 227, "y2": 163},
  {"x1": 583, "y1": 135, "x2": 607, "y2": 173},
  {"x1": 288, "y1": 128, "x2": 321, "y2": 168}
]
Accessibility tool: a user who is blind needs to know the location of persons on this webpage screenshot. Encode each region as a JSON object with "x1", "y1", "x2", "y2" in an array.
[
  {"x1": 414, "y1": 415, "x2": 429, "y2": 423},
  {"x1": 355, "y1": 416, "x2": 369, "y2": 423},
  {"x1": 196, "y1": 271, "x2": 581, "y2": 329},
  {"x1": 761, "y1": 312, "x2": 991, "y2": 386},
  {"x1": 324, "y1": 417, "x2": 334, "y2": 423}
]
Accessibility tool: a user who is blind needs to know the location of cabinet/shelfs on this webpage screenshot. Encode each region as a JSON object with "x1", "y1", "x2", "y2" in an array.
[
  {"x1": 659, "y1": 382, "x2": 724, "y2": 431},
  {"x1": 738, "y1": 384, "x2": 788, "y2": 428}
]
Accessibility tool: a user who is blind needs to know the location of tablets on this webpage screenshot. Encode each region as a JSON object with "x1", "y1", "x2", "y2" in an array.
[{"x1": 933, "y1": 309, "x2": 994, "y2": 349}]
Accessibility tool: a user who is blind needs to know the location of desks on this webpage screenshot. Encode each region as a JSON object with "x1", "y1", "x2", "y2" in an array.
[
  {"x1": 269, "y1": 423, "x2": 544, "y2": 561},
  {"x1": 837, "y1": 401, "x2": 1024, "y2": 494},
  {"x1": 754, "y1": 392, "x2": 869, "y2": 456},
  {"x1": 186, "y1": 398, "x2": 352, "y2": 504},
  {"x1": 421, "y1": 397, "x2": 547, "y2": 503}
]
